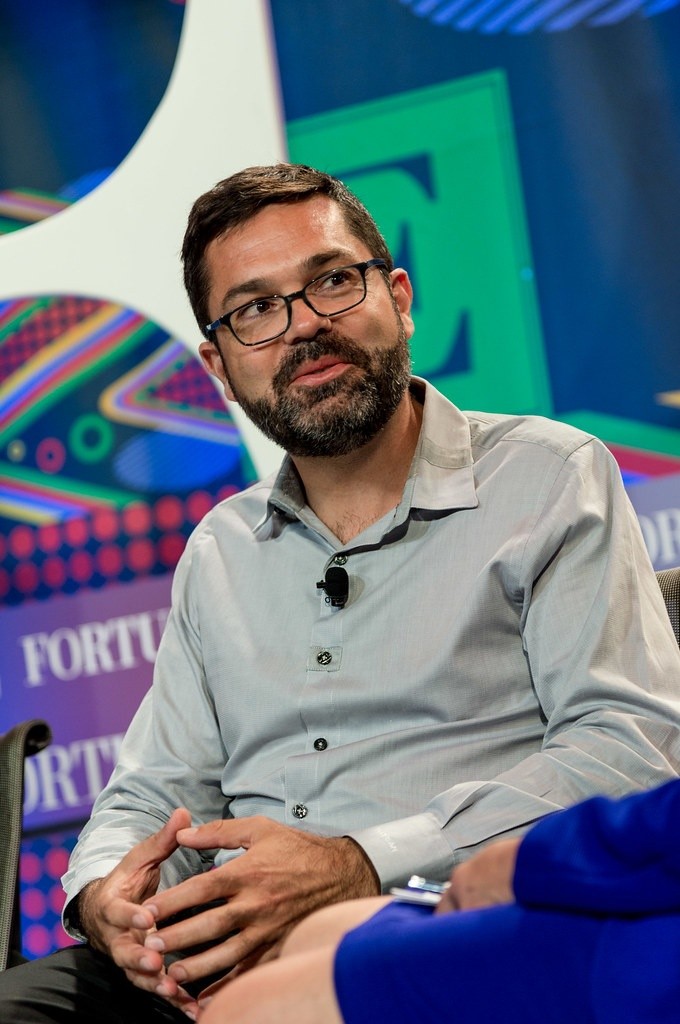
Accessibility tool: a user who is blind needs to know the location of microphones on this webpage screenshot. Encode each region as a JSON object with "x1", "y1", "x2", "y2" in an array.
[{"x1": 316, "y1": 567, "x2": 349, "y2": 610}]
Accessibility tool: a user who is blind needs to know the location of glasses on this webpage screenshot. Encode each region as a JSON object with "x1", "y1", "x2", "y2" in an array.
[{"x1": 205, "y1": 259, "x2": 389, "y2": 347}]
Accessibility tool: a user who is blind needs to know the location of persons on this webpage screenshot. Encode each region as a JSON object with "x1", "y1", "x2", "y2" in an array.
[
  {"x1": 0, "y1": 163, "x2": 680, "y2": 1024},
  {"x1": 196, "y1": 775, "x2": 680, "y2": 1024}
]
